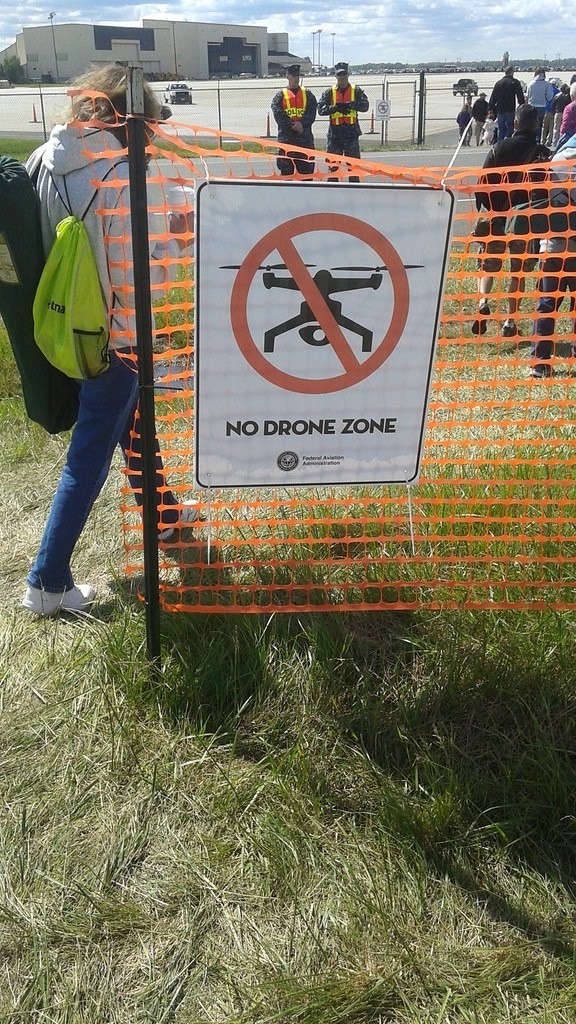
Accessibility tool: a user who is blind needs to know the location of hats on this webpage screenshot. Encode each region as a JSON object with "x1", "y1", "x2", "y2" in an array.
[
  {"x1": 335, "y1": 62, "x2": 348, "y2": 74},
  {"x1": 479, "y1": 92, "x2": 487, "y2": 96},
  {"x1": 284, "y1": 65, "x2": 305, "y2": 77}
]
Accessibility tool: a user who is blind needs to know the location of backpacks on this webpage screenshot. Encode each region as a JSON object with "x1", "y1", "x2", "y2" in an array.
[{"x1": 32, "y1": 159, "x2": 131, "y2": 381}]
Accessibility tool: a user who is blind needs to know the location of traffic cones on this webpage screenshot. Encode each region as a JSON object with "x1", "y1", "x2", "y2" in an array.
[{"x1": 33, "y1": 103, "x2": 37, "y2": 122}]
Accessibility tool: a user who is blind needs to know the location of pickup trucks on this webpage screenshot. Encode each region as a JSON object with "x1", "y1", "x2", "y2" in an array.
[{"x1": 453, "y1": 78, "x2": 478, "y2": 96}]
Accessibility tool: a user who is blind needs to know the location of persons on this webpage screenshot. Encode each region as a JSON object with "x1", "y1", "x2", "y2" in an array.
[
  {"x1": 317, "y1": 62, "x2": 370, "y2": 182},
  {"x1": 22, "y1": 65, "x2": 202, "y2": 616},
  {"x1": 271, "y1": 65, "x2": 318, "y2": 181},
  {"x1": 457, "y1": 65, "x2": 575, "y2": 377}
]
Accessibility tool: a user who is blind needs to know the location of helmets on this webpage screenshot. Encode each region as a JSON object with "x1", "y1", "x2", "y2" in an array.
[{"x1": 549, "y1": 77, "x2": 560, "y2": 88}]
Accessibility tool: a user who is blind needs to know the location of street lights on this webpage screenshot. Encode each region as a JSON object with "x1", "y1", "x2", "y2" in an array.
[
  {"x1": 317, "y1": 29, "x2": 323, "y2": 65},
  {"x1": 47, "y1": 11, "x2": 61, "y2": 84},
  {"x1": 310, "y1": 32, "x2": 317, "y2": 65},
  {"x1": 330, "y1": 33, "x2": 336, "y2": 66}
]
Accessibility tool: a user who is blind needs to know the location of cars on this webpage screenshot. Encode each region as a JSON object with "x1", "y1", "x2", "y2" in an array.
[
  {"x1": 211, "y1": 65, "x2": 576, "y2": 80},
  {"x1": 519, "y1": 80, "x2": 528, "y2": 93}
]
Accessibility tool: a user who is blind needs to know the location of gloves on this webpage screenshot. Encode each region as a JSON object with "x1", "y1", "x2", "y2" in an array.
[{"x1": 335, "y1": 101, "x2": 351, "y2": 115}]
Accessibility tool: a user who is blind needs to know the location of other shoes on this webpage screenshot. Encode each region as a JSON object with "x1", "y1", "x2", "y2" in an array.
[{"x1": 529, "y1": 368, "x2": 556, "y2": 378}]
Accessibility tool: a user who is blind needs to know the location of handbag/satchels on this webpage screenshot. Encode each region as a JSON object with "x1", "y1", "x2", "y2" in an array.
[
  {"x1": 557, "y1": 128, "x2": 574, "y2": 149},
  {"x1": 1, "y1": 154, "x2": 78, "y2": 434},
  {"x1": 471, "y1": 202, "x2": 536, "y2": 264},
  {"x1": 504, "y1": 191, "x2": 576, "y2": 240}
]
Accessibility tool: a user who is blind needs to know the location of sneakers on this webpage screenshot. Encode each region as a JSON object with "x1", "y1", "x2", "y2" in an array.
[
  {"x1": 501, "y1": 323, "x2": 522, "y2": 337},
  {"x1": 472, "y1": 302, "x2": 491, "y2": 334},
  {"x1": 21, "y1": 584, "x2": 93, "y2": 616},
  {"x1": 157, "y1": 500, "x2": 199, "y2": 540}
]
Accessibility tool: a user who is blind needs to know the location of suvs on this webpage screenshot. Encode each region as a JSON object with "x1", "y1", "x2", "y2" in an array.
[{"x1": 163, "y1": 83, "x2": 193, "y2": 105}]
